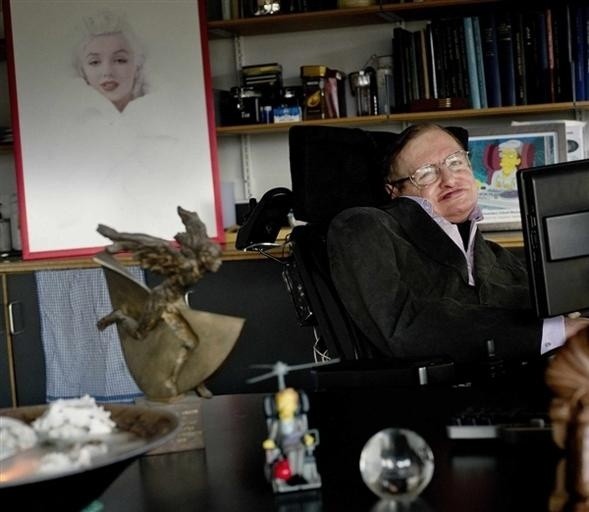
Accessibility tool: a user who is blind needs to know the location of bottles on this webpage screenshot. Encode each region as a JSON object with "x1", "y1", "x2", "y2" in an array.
[{"x1": 377, "y1": 53, "x2": 395, "y2": 115}]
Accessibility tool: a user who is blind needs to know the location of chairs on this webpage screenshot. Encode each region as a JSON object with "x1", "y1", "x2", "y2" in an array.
[{"x1": 231, "y1": 122, "x2": 475, "y2": 369}]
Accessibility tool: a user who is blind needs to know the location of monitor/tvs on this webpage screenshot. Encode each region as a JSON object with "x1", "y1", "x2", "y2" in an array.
[{"x1": 515, "y1": 160, "x2": 589, "y2": 319}]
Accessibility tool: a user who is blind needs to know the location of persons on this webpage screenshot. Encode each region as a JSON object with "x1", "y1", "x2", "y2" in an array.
[
  {"x1": 50, "y1": 14, "x2": 190, "y2": 247},
  {"x1": 328, "y1": 122, "x2": 589, "y2": 358},
  {"x1": 94, "y1": 204, "x2": 223, "y2": 402},
  {"x1": 490, "y1": 141, "x2": 524, "y2": 189}
]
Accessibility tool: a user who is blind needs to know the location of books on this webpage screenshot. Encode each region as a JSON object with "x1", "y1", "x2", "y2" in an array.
[{"x1": 389, "y1": 6, "x2": 588, "y2": 106}]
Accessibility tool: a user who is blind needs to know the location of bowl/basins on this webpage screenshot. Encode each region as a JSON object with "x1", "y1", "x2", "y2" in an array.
[{"x1": 0, "y1": 401, "x2": 183, "y2": 510}]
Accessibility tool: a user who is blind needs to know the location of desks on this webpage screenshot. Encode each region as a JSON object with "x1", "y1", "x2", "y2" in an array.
[{"x1": 0, "y1": 387, "x2": 589, "y2": 511}]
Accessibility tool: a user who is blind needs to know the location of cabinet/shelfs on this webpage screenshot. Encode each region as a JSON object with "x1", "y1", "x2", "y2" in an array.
[
  {"x1": 1, "y1": 271, "x2": 146, "y2": 406},
  {"x1": 0, "y1": 0, "x2": 589, "y2": 138}
]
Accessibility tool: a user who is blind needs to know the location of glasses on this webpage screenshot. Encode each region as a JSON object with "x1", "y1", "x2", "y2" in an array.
[{"x1": 392, "y1": 150, "x2": 471, "y2": 188}]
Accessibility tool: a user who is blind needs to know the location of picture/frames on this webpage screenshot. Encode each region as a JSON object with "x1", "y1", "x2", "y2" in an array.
[
  {"x1": 0, "y1": 0, "x2": 228, "y2": 264},
  {"x1": 465, "y1": 121, "x2": 570, "y2": 233}
]
